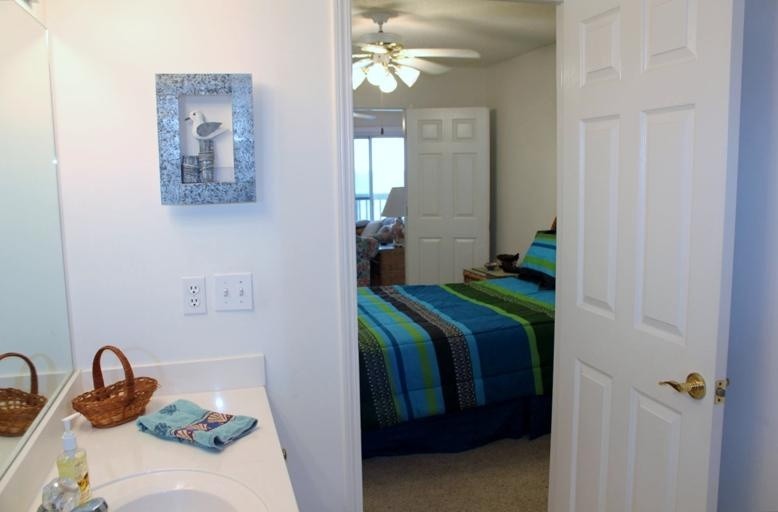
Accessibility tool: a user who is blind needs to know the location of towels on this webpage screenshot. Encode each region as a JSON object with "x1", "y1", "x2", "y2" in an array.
[{"x1": 136, "y1": 398, "x2": 258, "y2": 451}]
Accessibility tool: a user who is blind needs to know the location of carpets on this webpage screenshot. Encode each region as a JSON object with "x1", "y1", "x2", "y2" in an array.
[{"x1": 358, "y1": 439, "x2": 550, "y2": 511}]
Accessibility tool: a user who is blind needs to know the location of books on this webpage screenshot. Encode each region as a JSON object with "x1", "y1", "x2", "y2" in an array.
[{"x1": 463, "y1": 267, "x2": 519, "y2": 278}]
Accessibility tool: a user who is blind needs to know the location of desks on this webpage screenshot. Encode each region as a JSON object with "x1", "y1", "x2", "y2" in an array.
[{"x1": 370, "y1": 241, "x2": 406, "y2": 286}]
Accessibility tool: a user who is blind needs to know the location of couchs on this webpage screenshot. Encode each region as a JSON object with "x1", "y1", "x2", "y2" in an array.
[{"x1": 357, "y1": 221, "x2": 392, "y2": 245}]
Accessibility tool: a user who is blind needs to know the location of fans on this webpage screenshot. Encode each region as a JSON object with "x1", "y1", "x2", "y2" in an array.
[{"x1": 350, "y1": 2, "x2": 480, "y2": 75}]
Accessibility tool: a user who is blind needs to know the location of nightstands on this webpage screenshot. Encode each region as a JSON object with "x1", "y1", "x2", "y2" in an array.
[{"x1": 463, "y1": 262, "x2": 518, "y2": 284}]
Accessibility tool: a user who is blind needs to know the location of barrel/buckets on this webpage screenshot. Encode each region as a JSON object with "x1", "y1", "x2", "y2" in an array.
[{"x1": 80, "y1": 468, "x2": 270, "y2": 512}]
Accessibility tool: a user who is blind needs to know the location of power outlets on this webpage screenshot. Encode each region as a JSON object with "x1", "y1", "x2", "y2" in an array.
[{"x1": 182, "y1": 276, "x2": 205, "y2": 314}]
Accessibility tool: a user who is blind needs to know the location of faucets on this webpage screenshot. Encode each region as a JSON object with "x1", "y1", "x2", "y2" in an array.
[{"x1": 38, "y1": 476, "x2": 108, "y2": 512}]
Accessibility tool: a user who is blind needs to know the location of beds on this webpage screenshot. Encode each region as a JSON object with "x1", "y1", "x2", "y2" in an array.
[{"x1": 357, "y1": 217, "x2": 557, "y2": 458}]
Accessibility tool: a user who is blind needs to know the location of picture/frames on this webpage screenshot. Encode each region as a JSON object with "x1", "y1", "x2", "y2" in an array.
[{"x1": 156, "y1": 71, "x2": 258, "y2": 204}]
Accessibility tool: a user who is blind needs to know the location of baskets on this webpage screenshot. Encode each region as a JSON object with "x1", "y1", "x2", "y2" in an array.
[
  {"x1": 72, "y1": 345, "x2": 159, "y2": 431},
  {"x1": 0, "y1": 351, "x2": 49, "y2": 439}
]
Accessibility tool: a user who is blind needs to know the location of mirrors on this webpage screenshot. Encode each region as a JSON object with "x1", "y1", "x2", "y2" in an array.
[{"x1": 0, "y1": 1, "x2": 74, "y2": 479}]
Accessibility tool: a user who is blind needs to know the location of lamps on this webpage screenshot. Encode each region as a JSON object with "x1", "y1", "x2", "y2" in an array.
[
  {"x1": 380, "y1": 186, "x2": 407, "y2": 248},
  {"x1": 351, "y1": 53, "x2": 420, "y2": 94}
]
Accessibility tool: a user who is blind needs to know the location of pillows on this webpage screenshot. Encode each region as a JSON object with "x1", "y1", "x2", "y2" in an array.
[
  {"x1": 362, "y1": 222, "x2": 381, "y2": 237},
  {"x1": 374, "y1": 225, "x2": 392, "y2": 241}
]
